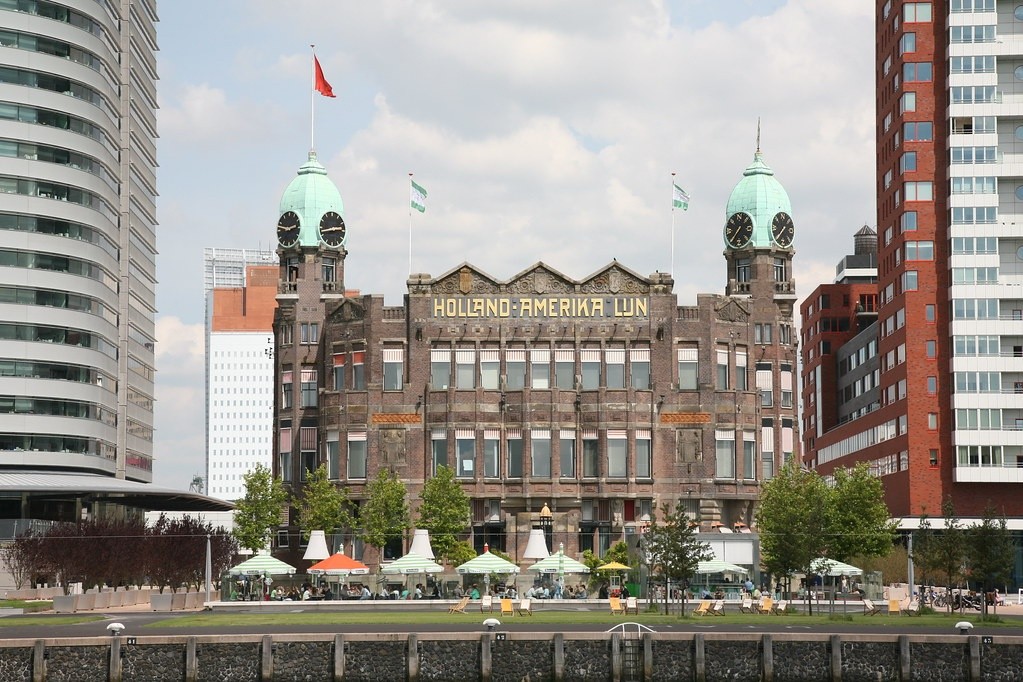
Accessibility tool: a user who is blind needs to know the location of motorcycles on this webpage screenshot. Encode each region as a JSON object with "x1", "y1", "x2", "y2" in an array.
[{"x1": 951, "y1": 591, "x2": 981, "y2": 611}]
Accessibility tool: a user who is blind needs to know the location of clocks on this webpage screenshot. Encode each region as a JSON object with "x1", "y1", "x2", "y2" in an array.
[
  {"x1": 773, "y1": 213, "x2": 795, "y2": 246},
  {"x1": 726, "y1": 212, "x2": 753, "y2": 248},
  {"x1": 319, "y1": 212, "x2": 347, "y2": 248},
  {"x1": 277, "y1": 213, "x2": 300, "y2": 246}
]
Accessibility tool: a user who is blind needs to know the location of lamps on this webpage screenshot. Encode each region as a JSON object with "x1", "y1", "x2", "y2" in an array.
[
  {"x1": 761, "y1": 346, "x2": 765, "y2": 354},
  {"x1": 307, "y1": 344, "x2": 310, "y2": 353},
  {"x1": 264, "y1": 337, "x2": 278, "y2": 359},
  {"x1": 660, "y1": 394, "x2": 665, "y2": 401},
  {"x1": 638, "y1": 326, "x2": 642, "y2": 332},
  {"x1": 439, "y1": 327, "x2": 443, "y2": 331},
  {"x1": 589, "y1": 327, "x2": 593, "y2": 332},
  {"x1": 790, "y1": 348, "x2": 793, "y2": 351},
  {"x1": 489, "y1": 326, "x2": 493, "y2": 332},
  {"x1": 563, "y1": 326, "x2": 567, "y2": 331},
  {"x1": 539, "y1": 323, "x2": 542, "y2": 333},
  {"x1": 614, "y1": 324, "x2": 618, "y2": 331},
  {"x1": 418, "y1": 394, "x2": 423, "y2": 402},
  {"x1": 514, "y1": 326, "x2": 517, "y2": 333}
]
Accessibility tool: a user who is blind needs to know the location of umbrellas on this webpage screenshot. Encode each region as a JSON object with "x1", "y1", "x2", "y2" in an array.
[
  {"x1": 229, "y1": 554, "x2": 297, "y2": 596},
  {"x1": 673, "y1": 556, "x2": 749, "y2": 586},
  {"x1": 381, "y1": 552, "x2": 444, "y2": 597},
  {"x1": 526, "y1": 551, "x2": 590, "y2": 573},
  {"x1": 455, "y1": 549, "x2": 520, "y2": 596},
  {"x1": 789, "y1": 557, "x2": 864, "y2": 599},
  {"x1": 307, "y1": 552, "x2": 370, "y2": 600},
  {"x1": 596, "y1": 561, "x2": 631, "y2": 586}
]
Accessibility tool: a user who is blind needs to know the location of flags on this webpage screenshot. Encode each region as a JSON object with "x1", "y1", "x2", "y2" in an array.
[
  {"x1": 410, "y1": 180, "x2": 427, "y2": 213},
  {"x1": 313, "y1": 52, "x2": 337, "y2": 98},
  {"x1": 672, "y1": 183, "x2": 689, "y2": 211}
]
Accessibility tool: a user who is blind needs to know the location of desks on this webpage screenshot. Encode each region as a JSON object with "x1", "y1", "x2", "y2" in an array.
[
  {"x1": 250, "y1": 594, "x2": 258, "y2": 601},
  {"x1": 310, "y1": 595, "x2": 325, "y2": 601},
  {"x1": 422, "y1": 594, "x2": 436, "y2": 600},
  {"x1": 461, "y1": 594, "x2": 473, "y2": 599},
  {"x1": 537, "y1": 593, "x2": 545, "y2": 598},
  {"x1": 235, "y1": 594, "x2": 244, "y2": 601},
  {"x1": 282, "y1": 595, "x2": 292, "y2": 600},
  {"x1": 348, "y1": 595, "x2": 362, "y2": 599}
]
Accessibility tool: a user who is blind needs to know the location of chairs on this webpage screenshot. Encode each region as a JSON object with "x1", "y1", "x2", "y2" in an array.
[
  {"x1": 517, "y1": 599, "x2": 533, "y2": 616},
  {"x1": 739, "y1": 594, "x2": 789, "y2": 615},
  {"x1": 624, "y1": 596, "x2": 639, "y2": 615},
  {"x1": 500, "y1": 599, "x2": 514, "y2": 617},
  {"x1": 862, "y1": 598, "x2": 882, "y2": 616},
  {"x1": 906, "y1": 596, "x2": 922, "y2": 616},
  {"x1": 888, "y1": 600, "x2": 902, "y2": 616},
  {"x1": 480, "y1": 595, "x2": 493, "y2": 613},
  {"x1": 709, "y1": 600, "x2": 725, "y2": 616},
  {"x1": 608, "y1": 597, "x2": 626, "y2": 616},
  {"x1": 693, "y1": 600, "x2": 713, "y2": 616},
  {"x1": 448, "y1": 597, "x2": 471, "y2": 615}
]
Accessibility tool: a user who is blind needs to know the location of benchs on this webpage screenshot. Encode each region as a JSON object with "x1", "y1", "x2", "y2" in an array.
[{"x1": 985, "y1": 592, "x2": 1003, "y2": 606}]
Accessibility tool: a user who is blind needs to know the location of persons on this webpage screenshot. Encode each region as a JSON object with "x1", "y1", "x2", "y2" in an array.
[
  {"x1": 230, "y1": 576, "x2": 441, "y2": 600},
  {"x1": 599, "y1": 582, "x2": 630, "y2": 599},
  {"x1": 994, "y1": 589, "x2": 1005, "y2": 606},
  {"x1": 702, "y1": 577, "x2": 783, "y2": 599},
  {"x1": 454, "y1": 584, "x2": 587, "y2": 599},
  {"x1": 797, "y1": 575, "x2": 867, "y2": 600}
]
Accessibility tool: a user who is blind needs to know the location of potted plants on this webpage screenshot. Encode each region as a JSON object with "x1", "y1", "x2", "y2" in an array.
[{"x1": 0, "y1": 511, "x2": 239, "y2": 611}]
